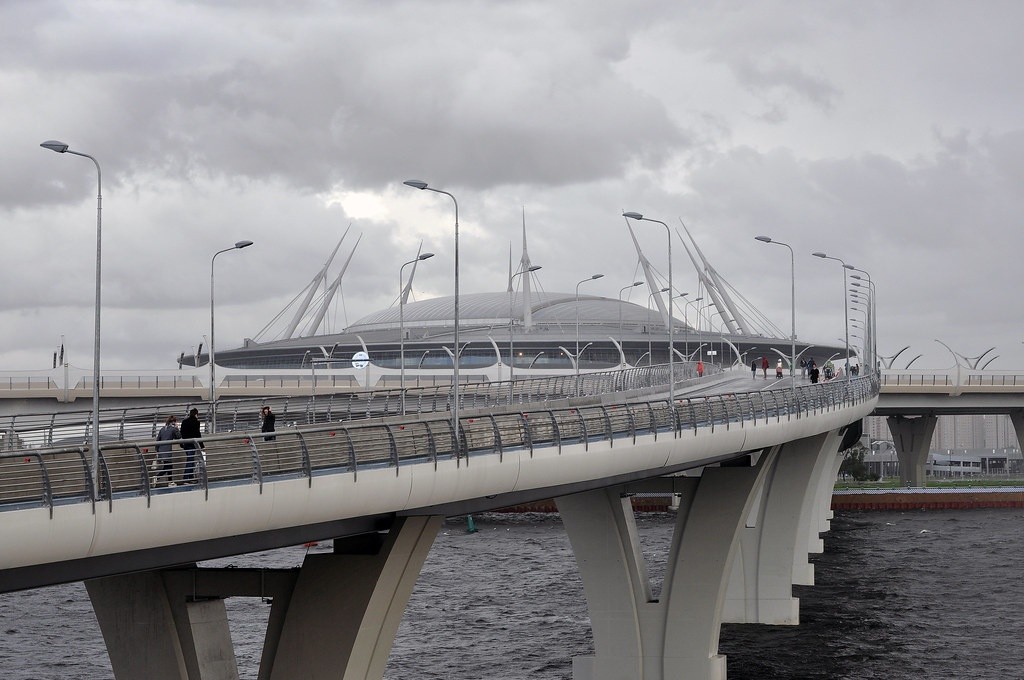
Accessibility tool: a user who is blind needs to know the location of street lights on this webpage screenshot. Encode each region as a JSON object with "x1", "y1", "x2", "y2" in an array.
[
  {"x1": 404, "y1": 178, "x2": 460, "y2": 460},
  {"x1": 647, "y1": 287, "x2": 669, "y2": 387},
  {"x1": 812, "y1": 250, "x2": 853, "y2": 407},
  {"x1": 844, "y1": 263, "x2": 879, "y2": 385},
  {"x1": 621, "y1": 210, "x2": 677, "y2": 432},
  {"x1": 508, "y1": 265, "x2": 542, "y2": 406},
  {"x1": 39, "y1": 140, "x2": 104, "y2": 501},
  {"x1": 397, "y1": 251, "x2": 438, "y2": 417},
  {"x1": 210, "y1": 239, "x2": 255, "y2": 434},
  {"x1": 573, "y1": 271, "x2": 607, "y2": 401},
  {"x1": 619, "y1": 281, "x2": 642, "y2": 393},
  {"x1": 753, "y1": 233, "x2": 798, "y2": 415},
  {"x1": 670, "y1": 293, "x2": 742, "y2": 382}
]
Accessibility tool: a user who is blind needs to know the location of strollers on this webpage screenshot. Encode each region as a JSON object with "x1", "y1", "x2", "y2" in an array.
[{"x1": 774, "y1": 366, "x2": 784, "y2": 379}]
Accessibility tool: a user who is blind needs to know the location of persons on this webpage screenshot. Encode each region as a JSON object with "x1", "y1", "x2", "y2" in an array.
[
  {"x1": 697, "y1": 362, "x2": 703, "y2": 377},
  {"x1": 777, "y1": 359, "x2": 782, "y2": 369},
  {"x1": 800, "y1": 357, "x2": 859, "y2": 379},
  {"x1": 810, "y1": 363, "x2": 819, "y2": 384},
  {"x1": 152, "y1": 415, "x2": 182, "y2": 487},
  {"x1": 762, "y1": 356, "x2": 767, "y2": 378},
  {"x1": 752, "y1": 361, "x2": 756, "y2": 378},
  {"x1": 180, "y1": 408, "x2": 205, "y2": 485},
  {"x1": 262, "y1": 406, "x2": 276, "y2": 440}
]
